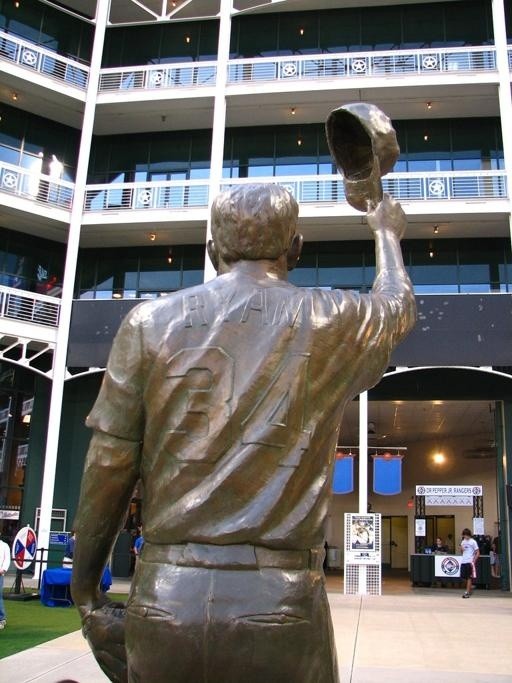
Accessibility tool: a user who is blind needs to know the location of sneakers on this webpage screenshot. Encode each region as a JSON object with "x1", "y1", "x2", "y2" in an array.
[{"x1": 462, "y1": 591, "x2": 472, "y2": 598}]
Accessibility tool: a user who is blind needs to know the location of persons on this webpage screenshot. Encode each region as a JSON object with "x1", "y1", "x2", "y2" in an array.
[
  {"x1": 433, "y1": 536, "x2": 447, "y2": 553},
  {"x1": 488, "y1": 531, "x2": 502, "y2": 578},
  {"x1": 62, "y1": 527, "x2": 75, "y2": 567},
  {"x1": 459, "y1": 528, "x2": 481, "y2": 597},
  {"x1": 71, "y1": 179, "x2": 420, "y2": 683},
  {"x1": 134, "y1": 523, "x2": 143, "y2": 566},
  {"x1": 0, "y1": 540, "x2": 11, "y2": 628},
  {"x1": 446, "y1": 533, "x2": 455, "y2": 552}
]
